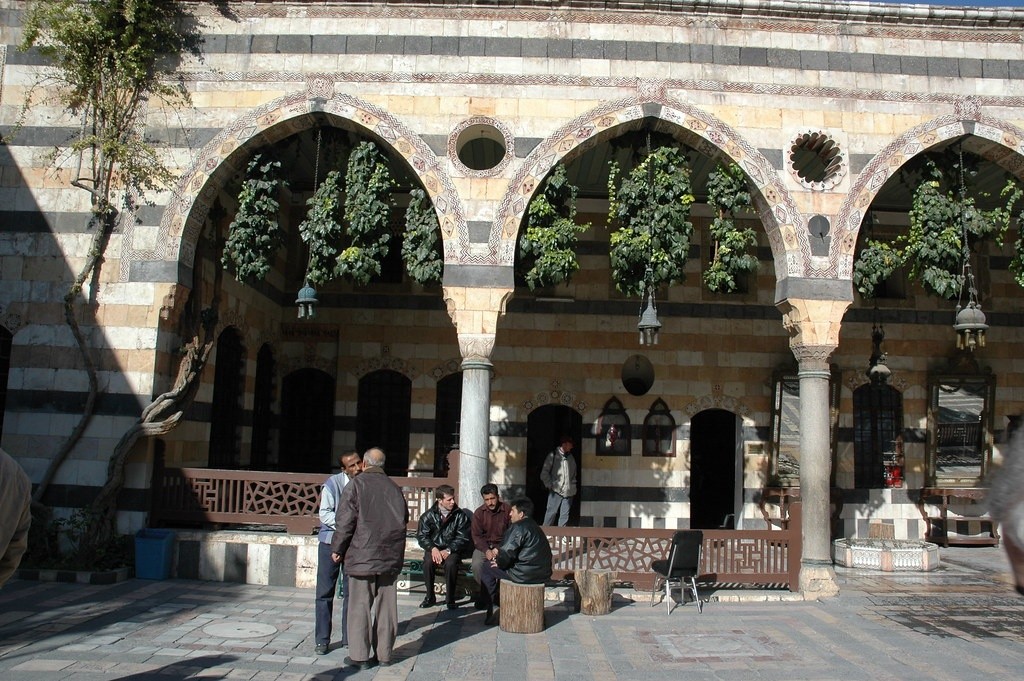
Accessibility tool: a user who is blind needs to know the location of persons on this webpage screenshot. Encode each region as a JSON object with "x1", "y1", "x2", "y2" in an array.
[
  {"x1": 480, "y1": 498, "x2": 554, "y2": 624},
  {"x1": 0, "y1": 449, "x2": 32, "y2": 587},
  {"x1": 417, "y1": 485, "x2": 471, "y2": 610},
  {"x1": 315, "y1": 451, "x2": 363, "y2": 654},
  {"x1": 471, "y1": 484, "x2": 512, "y2": 610},
  {"x1": 331, "y1": 448, "x2": 409, "y2": 670},
  {"x1": 540, "y1": 436, "x2": 577, "y2": 545}
]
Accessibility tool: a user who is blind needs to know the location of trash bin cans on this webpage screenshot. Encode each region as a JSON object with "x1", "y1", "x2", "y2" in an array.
[{"x1": 134, "y1": 529, "x2": 175, "y2": 580}]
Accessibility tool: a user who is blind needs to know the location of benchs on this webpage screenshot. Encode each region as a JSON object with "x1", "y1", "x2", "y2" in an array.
[{"x1": 402, "y1": 560, "x2": 472, "y2": 594}]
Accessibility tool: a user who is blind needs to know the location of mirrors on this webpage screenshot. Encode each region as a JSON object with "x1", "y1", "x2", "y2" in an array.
[{"x1": 926, "y1": 371, "x2": 998, "y2": 487}]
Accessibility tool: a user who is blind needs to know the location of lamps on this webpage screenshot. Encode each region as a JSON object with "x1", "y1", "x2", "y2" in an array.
[
  {"x1": 638, "y1": 131, "x2": 662, "y2": 345},
  {"x1": 296, "y1": 129, "x2": 322, "y2": 321},
  {"x1": 954, "y1": 143, "x2": 988, "y2": 349}
]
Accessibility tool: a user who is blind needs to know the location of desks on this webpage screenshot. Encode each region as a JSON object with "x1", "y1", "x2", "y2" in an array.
[{"x1": 920, "y1": 487, "x2": 1001, "y2": 547}]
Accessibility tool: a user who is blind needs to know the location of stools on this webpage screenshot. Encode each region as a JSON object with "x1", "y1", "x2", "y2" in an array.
[
  {"x1": 574, "y1": 568, "x2": 612, "y2": 615},
  {"x1": 499, "y1": 578, "x2": 545, "y2": 634}
]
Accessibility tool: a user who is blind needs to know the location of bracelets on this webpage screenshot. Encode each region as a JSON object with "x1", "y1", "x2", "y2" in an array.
[{"x1": 446, "y1": 549, "x2": 451, "y2": 554}]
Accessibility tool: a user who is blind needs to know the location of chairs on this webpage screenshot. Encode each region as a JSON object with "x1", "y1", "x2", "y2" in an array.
[{"x1": 651, "y1": 530, "x2": 704, "y2": 616}]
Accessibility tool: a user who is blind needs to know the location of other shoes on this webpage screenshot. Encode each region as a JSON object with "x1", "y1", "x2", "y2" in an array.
[
  {"x1": 377, "y1": 660, "x2": 389, "y2": 666},
  {"x1": 446, "y1": 601, "x2": 458, "y2": 608},
  {"x1": 315, "y1": 642, "x2": 327, "y2": 655},
  {"x1": 420, "y1": 597, "x2": 435, "y2": 609},
  {"x1": 344, "y1": 656, "x2": 368, "y2": 670},
  {"x1": 486, "y1": 604, "x2": 500, "y2": 626}
]
